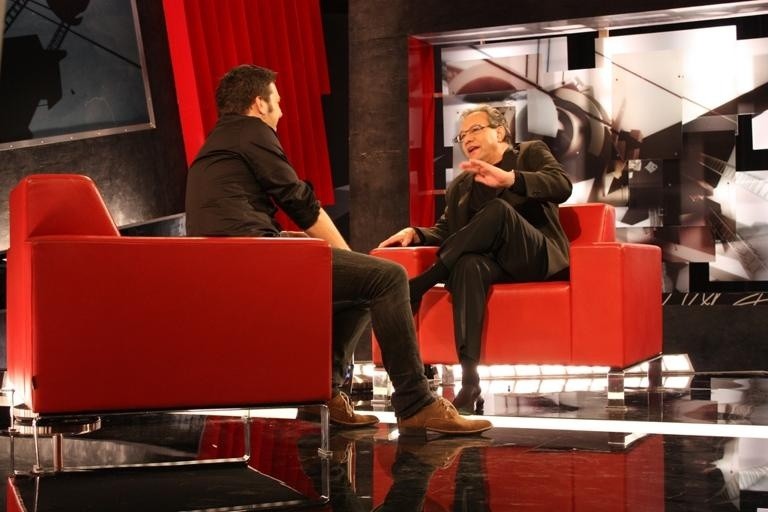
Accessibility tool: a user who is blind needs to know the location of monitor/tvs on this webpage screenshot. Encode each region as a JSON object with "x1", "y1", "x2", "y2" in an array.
[{"x1": 431, "y1": 13, "x2": 768, "y2": 307}]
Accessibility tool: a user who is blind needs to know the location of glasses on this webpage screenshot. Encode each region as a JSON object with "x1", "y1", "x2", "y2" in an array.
[{"x1": 454, "y1": 124, "x2": 490, "y2": 143}]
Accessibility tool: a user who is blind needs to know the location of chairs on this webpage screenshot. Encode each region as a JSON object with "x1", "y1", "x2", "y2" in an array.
[
  {"x1": 368, "y1": 202, "x2": 662, "y2": 412},
  {"x1": 6, "y1": 173, "x2": 334, "y2": 474}
]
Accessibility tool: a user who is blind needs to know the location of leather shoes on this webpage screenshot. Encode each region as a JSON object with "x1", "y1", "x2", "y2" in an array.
[
  {"x1": 452, "y1": 385, "x2": 480, "y2": 413},
  {"x1": 396, "y1": 437, "x2": 491, "y2": 469},
  {"x1": 297, "y1": 430, "x2": 377, "y2": 465},
  {"x1": 398, "y1": 397, "x2": 492, "y2": 436},
  {"x1": 296, "y1": 393, "x2": 380, "y2": 427}
]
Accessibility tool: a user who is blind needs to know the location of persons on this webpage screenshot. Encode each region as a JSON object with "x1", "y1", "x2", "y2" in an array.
[
  {"x1": 298, "y1": 422, "x2": 495, "y2": 511},
  {"x1": 185, "y1": 64, "x2": 493, "y2": 437},
  {"x1": 378, "y1": 106, "x2": 572, "y2": 415},
  {"x1": 353, "y1": 439, "x2": 489, "y2": 509}
]
